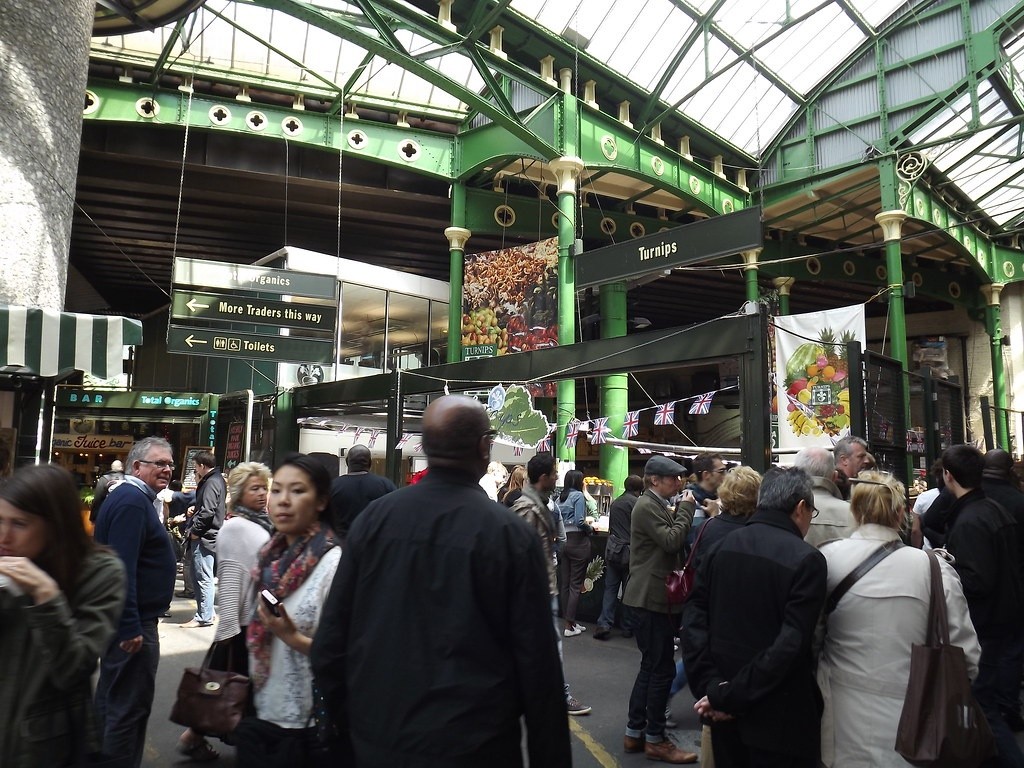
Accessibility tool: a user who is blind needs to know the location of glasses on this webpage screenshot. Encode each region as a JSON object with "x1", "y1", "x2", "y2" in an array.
[
  {"x1": 802, "y1": 496, "x2": 819, "y2": 518},
  {"x1": 483, "y1": 429, "x2": 499, "y2": 440},
  {"x1": 138, "y1": 459, "x2": 176, "y2": 470},
  {"x1": 698, "y1": 468, "x2": 728, "y2": 475}
]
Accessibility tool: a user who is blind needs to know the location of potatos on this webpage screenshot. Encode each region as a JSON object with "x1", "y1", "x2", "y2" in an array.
[{"x1": 463, "y1": 311, "x2": 509, "y2": 357}]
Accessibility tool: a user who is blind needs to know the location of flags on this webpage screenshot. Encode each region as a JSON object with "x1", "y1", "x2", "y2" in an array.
[
  {"x1": 689, "y1": 393, "x2": 713, "y2": 414},
  {"x1": 537, "y1": 437, "x2": 550, "y2": 453},
  {"x1": 394, "y1": 434, "x2": 413, "y2": 449},
  {"x1": 591, "y1": 418, "x2": 607, "y2": 445},
  {"x1": 367, "y1": 431, "x2": 381, "y2": 449},
  {"x1": 352, "y1": 427, "x2": 366, "y2": 444},
  {"x1": 622, "y1": 412, "x2": 639, "y2": 438},
  {"x1": 917, "y1": 434, "x2": 926, "y2": 453},
  {"x1": 654, "y1": 403, "x2": 675, "y2": 425},
  {"x1": 334, "y1": 424, "x2": 352, "y2": 437},
  {"x1": 566, "y1": 424, "x2": 578, "y2": 446},
  {"x1": 906, "y1": 432, "x2": 914, "y2": 452},
  {"x1": 513, "y1": 447, "x2": 523, "y2": 456}
]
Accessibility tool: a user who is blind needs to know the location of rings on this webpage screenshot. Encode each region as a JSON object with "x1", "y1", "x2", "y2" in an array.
[{"x1": 7, "y1": 565, "x2": 15, "y2": 570}]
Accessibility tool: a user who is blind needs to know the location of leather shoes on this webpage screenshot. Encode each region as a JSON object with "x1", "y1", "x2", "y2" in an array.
[
  {"x1": 624, "y1": 736, "x2": 645, "y2": 753},
  {"x1": 645, "y1": 737, "x2": 698, "y2": 763}
]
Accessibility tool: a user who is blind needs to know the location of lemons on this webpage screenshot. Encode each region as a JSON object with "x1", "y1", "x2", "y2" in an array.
[{"x1": 800, "y1": 419, "x2": 822, "y2": 436}]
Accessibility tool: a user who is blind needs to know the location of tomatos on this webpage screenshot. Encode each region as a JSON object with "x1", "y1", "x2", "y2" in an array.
[
  {"x1": 527, "y1": 382, "x2": 556, "y2": 398},
  {"x1": 509, "y1": 316, "x2": 557, "y2": 352}
]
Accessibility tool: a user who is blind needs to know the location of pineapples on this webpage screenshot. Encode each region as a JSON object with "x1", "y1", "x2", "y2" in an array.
[{"x1": 818, "y1": 326, "x2": 855, "y2": 371}]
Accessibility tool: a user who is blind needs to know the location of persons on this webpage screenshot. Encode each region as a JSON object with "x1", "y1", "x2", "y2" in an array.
[
  {"x1": 234, "y1": 453, "x2": 344, "y2": 768},
  {"x1": 89, "y1": 435, "x2": 1024, "y2": 768},
  {"x1": 1, "y1": 462, "x2": 127, "y2": 767},
  {"x1": 310, "y1": 394, "x2": 572, "y2": 767},
  {"x1": 680, "y1": 466, "x2": 830, "y2": 768}
]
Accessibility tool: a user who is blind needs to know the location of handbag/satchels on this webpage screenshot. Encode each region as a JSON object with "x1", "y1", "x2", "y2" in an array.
[
  {"x1": 168, "y1": 639, "x2": 258, "y2": 746},
  {"x1": 894, "y1": 554, "x2": 999, "y2": 768},
  {"x1": 665, "y1": 568, "x2": 694, "y2": 603}
]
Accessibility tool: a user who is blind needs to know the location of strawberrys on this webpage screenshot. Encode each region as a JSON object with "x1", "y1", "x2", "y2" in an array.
[{"x1": 836, "y1": 405, "x2": 844, "y2": 413}]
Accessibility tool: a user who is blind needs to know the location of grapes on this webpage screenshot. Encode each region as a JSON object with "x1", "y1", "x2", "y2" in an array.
[{"x1": 818, "y1": 404, "x2": 846, "y2": 430}]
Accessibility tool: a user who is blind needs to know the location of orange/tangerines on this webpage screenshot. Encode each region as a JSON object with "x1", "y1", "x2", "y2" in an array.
[{"x1": 805, "y1": 365, "x2": 835, "y2": 390}]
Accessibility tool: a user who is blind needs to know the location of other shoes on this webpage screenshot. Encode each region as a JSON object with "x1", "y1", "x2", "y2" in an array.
[
  {"x1": 564, "y1": 623, "x2": 586, "y2": 637},
  {"x1": 594, "y1": 628, "x2": 609, "y2": 638},
  {"x1": 176, "y1": 738, "x2": 221, "y2": 761},
  {"x1": 175, "y1": 590, "x2": 194, "y2": 599},
  {"x1": 179, "y1": 619, "x2": 211, "y2": 627},
  {"x1": 622, "y1": 631, "x2": 633, "y2": 638},
  {"x1": 665, "y1": 702, "x2": 680, "y2": 728},
  {"x1": 566, "y1": 698, "x2": 592, "y2": 715}
]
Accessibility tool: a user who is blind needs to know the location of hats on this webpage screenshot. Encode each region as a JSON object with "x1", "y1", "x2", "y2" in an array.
[{"x1": 644, "y1": 455, "x2": 687, "y2": 475}]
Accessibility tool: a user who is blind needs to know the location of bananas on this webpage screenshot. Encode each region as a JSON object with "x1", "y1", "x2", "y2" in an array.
[
  {"x1": 787, "y1": 411, "x2": 808, "y2": 437},
  {"x1": 837, "y1": 390, "x2": 850, "y2": 430}
]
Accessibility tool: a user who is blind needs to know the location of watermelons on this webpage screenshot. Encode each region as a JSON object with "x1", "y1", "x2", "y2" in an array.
[{"x1": 786, "y1": 342, "x2": 823, "y2": 375}]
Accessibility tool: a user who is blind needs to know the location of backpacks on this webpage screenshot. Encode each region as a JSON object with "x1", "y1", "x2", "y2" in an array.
[{"x1": 103, "y1": 474, "x2": 126, "y2": 500}]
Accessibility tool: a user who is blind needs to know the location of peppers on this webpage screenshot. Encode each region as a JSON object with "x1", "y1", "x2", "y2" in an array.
[{"x1": 789, "y1": 380, "x2": 813, "y2": 403}]
